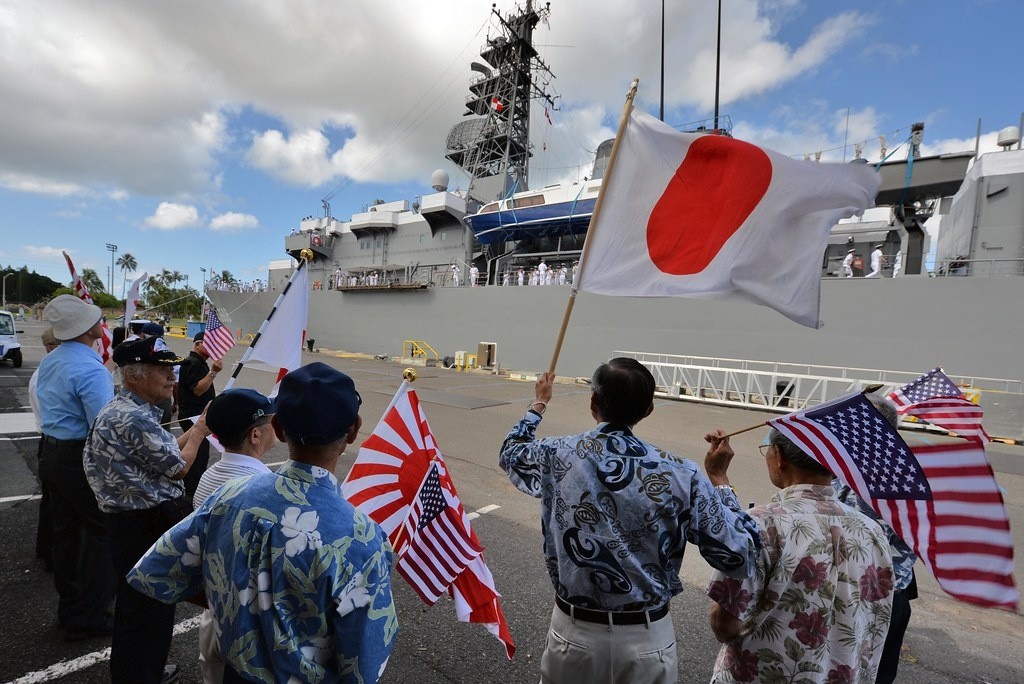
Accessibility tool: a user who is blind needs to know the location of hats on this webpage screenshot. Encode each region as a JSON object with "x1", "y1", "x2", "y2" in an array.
[
  {"x1": 875, "y1": 244, "x2": 883, "y2": 248},
  {"x1": 130, "y1": 320, "x2": 151, "y2": 335},
  {"x1": 112, "y1": 336, "x2": 185, "y2": 367},
  {"x1": 193, "y1": 332, "x2": 204, "y2": 343},
  {"x1": 848, "y1": 249, "x2": 855, "y2": 253},
  {"x1": 44, "y1": 294, "x2": 101, "y2": 341},
  {"x1": 141, "y1": 323, "x2": 165, "y2": 336},
  {"x1": 274, "y1": 362, "x2": 362, "y2": 447},
  {"x1": 205, "y1": 387, "x2": 277, "y2": 436},
  {"x1": 42, "y1": 327, "x2": 62, "y2": 346},
  {"x1": 337, "y1": 258, "x2": 581, "y2": 278}
]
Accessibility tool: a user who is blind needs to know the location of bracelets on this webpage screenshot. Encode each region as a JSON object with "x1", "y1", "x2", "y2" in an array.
[
  {"x1": 530, "y1": 401, "x2": 546, "y2": 415},
  {"x1": 713, "y1": 485, "x2": 732, "y2": 490}
]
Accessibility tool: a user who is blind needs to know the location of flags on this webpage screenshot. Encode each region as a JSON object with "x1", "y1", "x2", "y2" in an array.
[
  {"x1": 491, "y1": 98, "x2": 503, "y2": 111},
  {"x1": 242, "y1": 256, "x2": 310, "y2": 397},
  {"x1": 202, "y1": 309, "x2": 237, "y2": 363},
  {"x1": 544, "y1": 109, "x2": 554, "y2": 126},
  {"x1": 766, "y1": 369, "x2": 1024, "y2": 616},
  {"x1": 125, "y1": 272, "x2": 148, "y2": 327},
  {"x1": 341, "y1": 384, "x2": 517, "y2": 662},
  {"x1": 63, "y1": 256, "x2": 113, "y2": 364},
  {"x1": 577, "y1": 105, "x2": 882, "y2": 331},
  {"x1": 192, "y1": 415, "x2": 226, "y2": 454}
]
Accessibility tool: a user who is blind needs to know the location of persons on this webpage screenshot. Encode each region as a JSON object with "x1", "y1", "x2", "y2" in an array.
[
  {"x1": 134, "y1": 313, "x2": 171, "y2": 323},
  {"x1": 843, "y1": 244, "x2": 902, "y2": 279},
  {"x1": 290, "y1": 228, "x2": 295, "y2": 237},
  {"x1": 332, "y1": 216, "x2": 335, "y2": 219},
  {"x1": 187, "y1": 313, "x2": 193, "y2": 321},
  {"x1": 336, "y1": 267, "x2": 379, "y2": 287},
  {"x1": 499, "y1": 355, "x2": 763, "y2": 684},
  {"x1": 302, "y1": 215, "x2": 313, "y2": 221},
  {"x1": 205, "y1": 279, "x2": 270, "y2": 292},
  {"x1": 451, "y1": 263, "x2": 482, "y2": 287},
  {"x1": 501, "y1": 259, "x2": 578, "y2": 286},
  {"x1": 17, "y1": 304, "x2": 27, "y2": 322},
  {"x1": 707, "y1": 396, "x2": 918, "y2": 684},
  {"x1": 28, "y1": 297, "x2": 400, "y2": 684}
]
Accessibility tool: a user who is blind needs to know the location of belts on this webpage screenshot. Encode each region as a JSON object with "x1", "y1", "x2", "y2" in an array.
[
  {"x1": 553, "y1": 592, "x2": 670, "y2": 625},
  {"x1": 44, "y1": 435, "x2": 85, "y2": 446}
]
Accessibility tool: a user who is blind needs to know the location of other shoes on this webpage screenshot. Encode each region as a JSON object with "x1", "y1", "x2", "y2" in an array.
[
  {"x1": 58, "y1": 598, "x2": 71, "y2": 630},
  {"x1": 159, "y1": 663, "x2": 180, "y2": 684},
  {"x1": 63, "y1": 610, "x2": 114, "y2": 641},
  {"x1": 35, "y1": 549, "x2": 54, "y2": 572}
]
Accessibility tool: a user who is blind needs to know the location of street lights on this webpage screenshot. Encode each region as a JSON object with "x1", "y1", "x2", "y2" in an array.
[
  {"x1": 106, "y1": 243, "x2": 117, "y2": 314},
  {"x1": 3, "y1": 273, "x2": 14, "y2": 310},
  {"x1": 200, "y1": 266, "x2": 206, "y2": 293}
]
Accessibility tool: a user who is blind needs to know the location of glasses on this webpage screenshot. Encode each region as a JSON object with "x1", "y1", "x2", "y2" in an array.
[{"x1": 758, "y1": 441, "x2": 785, "y2": 458}]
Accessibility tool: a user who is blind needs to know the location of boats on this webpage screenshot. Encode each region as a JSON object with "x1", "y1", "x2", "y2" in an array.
[
  {"x1": 204, "y1": 0, "x2": 1024, "y2": 441},
  {"x1": 463, "y1": 178, "x2": 603, "y2": 244}
]
